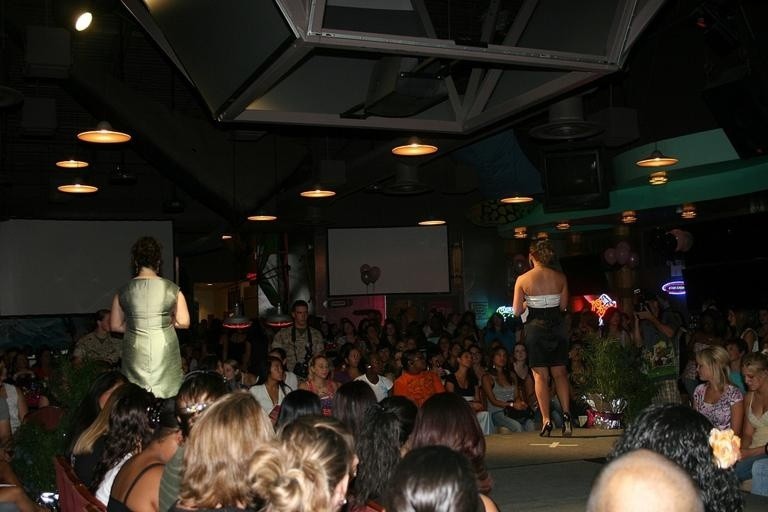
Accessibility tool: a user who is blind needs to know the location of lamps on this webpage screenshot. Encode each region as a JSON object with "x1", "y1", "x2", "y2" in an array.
[
  {"x1": 302, "y1": 184, "x2": 335, "y2": 197},
  {"x1": 514, "y1": 206, "x2": 696, "y2": 237},
  {"x1": 57, "y1": 157, "x2": 88, "y2": 169},
  {"x1": 636, "y1": 152, "x2": 679, "y2": 166},
  {"x1": 248, "y1": 210, "x2": 276, "y2": 221},
  {"x1": 419, "y1": 217, "x2": 445, "y2": 226},
  {"x1": 501, "y1": 193, "x2": 532, "y2": 203},
  {"x1": 58, "y1": 179, "x2": 98, "y2": 193},
  {"x1": 391, "y1": 137, "x2": 437, "y2": 155},
  {"x1": 650, "y1": 173, "x2": 670, "y2": 185},
  {"x1": 77, "y1": 121, "x2": 132, "y2": 144}
]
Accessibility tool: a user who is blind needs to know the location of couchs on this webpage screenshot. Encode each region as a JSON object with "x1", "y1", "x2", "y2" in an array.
[{"x1": 1, "y1": 313, "x2": 98, "y2": 356}]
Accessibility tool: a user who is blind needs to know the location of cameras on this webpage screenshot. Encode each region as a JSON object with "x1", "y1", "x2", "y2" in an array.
[
  {"x1": 634, "y1": 298, "x2": 650, "y2": 311},
  {"x1": 294, "y1": 362, "x2": 309, "y2": 379}
]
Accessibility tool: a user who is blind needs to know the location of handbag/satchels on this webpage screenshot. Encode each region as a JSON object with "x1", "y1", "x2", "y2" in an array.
[{"x1": 505, "y1": 400, "x2": 535, "y2": 423}]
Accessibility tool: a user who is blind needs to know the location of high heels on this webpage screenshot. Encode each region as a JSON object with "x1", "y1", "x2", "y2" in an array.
[
  {"x1": 540, "y1": 422, "x2": 553, "y2": 437},
  {"x1": 562, "y1": 412, "x2": 572, "y2": 437}
]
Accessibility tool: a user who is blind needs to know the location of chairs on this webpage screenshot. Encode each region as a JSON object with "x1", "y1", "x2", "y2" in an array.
[{"x1": 54, "y1": 459, "x2": 106, "y2": 512}]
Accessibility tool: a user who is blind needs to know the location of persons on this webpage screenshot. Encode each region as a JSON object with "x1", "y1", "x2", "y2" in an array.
[
  {"x1": 1, "y1": 301, "x2": 768, "y2": 511},
  {"x1": 110, "y1": 237, "x2": 189, "y2": 410},
  {"x1": 512, "y1": 233, "x2": 572, "y2": 438}
]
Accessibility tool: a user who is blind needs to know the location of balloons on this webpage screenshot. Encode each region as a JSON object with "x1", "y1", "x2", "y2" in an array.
[
  {"x1": 360, "y1": 265, "x2": 380, "y2": 285},
  {"x1": 605, "y1": 242, "x2": 639, "y2": 271},
  {"x1": 513, "y1": 254, "x2": 528, "y2": 274},
  {"x1": 656, "y1": 229, "x2": 693, "y2": 252}
]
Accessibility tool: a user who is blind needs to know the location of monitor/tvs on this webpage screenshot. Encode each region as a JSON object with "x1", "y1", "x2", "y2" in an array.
[{"x1": 542, "y1": 139, "x2": 609, "y2": 213}]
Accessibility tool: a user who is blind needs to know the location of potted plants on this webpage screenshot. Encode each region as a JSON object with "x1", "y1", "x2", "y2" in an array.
[{"x1": 572, "y1": 333, "x2": 659, "y2": 430}]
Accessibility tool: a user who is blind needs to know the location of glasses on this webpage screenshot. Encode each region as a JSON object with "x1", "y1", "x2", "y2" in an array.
[{"x1": 184, "y1": 369, "x2": 227, "y2": 382}]
